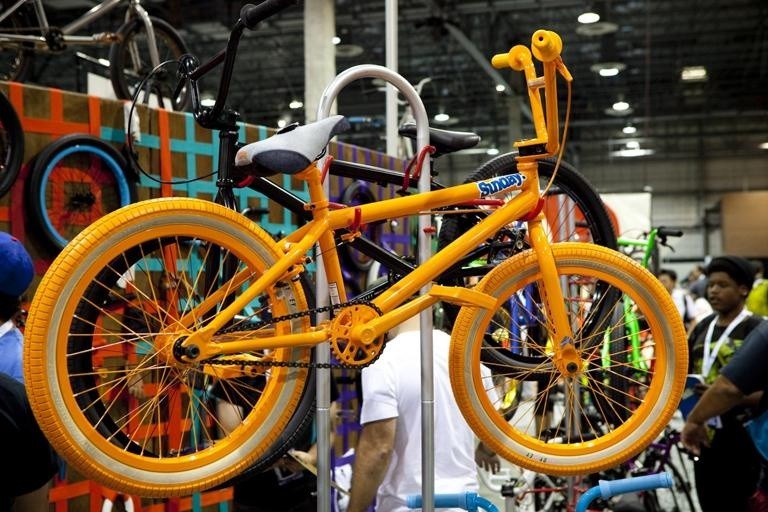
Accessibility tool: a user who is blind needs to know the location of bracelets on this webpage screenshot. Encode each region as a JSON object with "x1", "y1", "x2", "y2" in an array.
[{"x1": 478, "y1": 441, "x2": 496, "y2": 457}]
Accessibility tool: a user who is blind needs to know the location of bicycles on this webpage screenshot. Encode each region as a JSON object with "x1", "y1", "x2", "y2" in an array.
[
  {"x1": 66, "y1": 0, "x2": 624, "y2": 494},
  {"x1": 20, "y1": 28, "x2": 691, "y2": 499},
  {"x1": 1, "y1": 0, "x2": 197, "y2": 112}
]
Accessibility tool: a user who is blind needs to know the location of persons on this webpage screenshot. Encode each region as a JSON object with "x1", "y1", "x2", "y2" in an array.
[
  {"x1": 655, "y1": 253, "x2": 768, "y2": 512},
  {"x1": 347, "y1": 296, "x2": 502, "y2": 512},
  {"x1": 0, "y1": 230, "x2": 63, "y2": 512}
]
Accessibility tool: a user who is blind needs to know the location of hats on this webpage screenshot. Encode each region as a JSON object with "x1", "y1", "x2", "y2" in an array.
[{"x1": 710, "y1": 256, "x2": 756, "y2": 287}]
